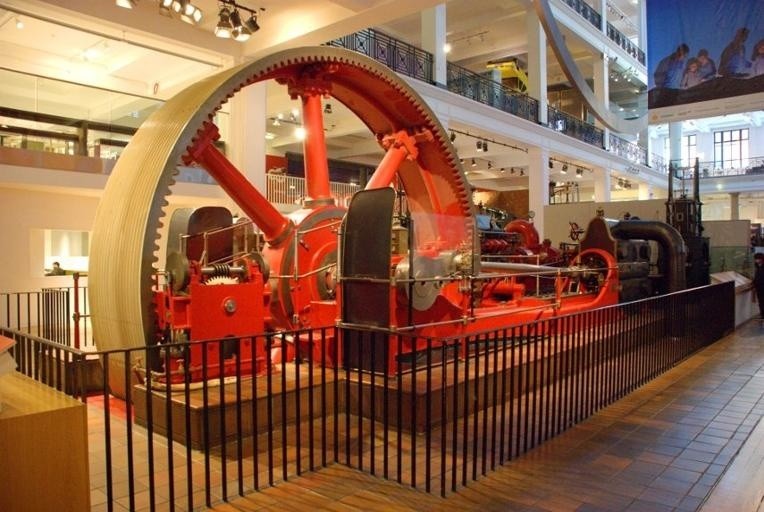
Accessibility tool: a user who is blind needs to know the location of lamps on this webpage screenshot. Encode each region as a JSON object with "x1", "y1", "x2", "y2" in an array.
[
  {"x1": 548, "y1": 160, "x2": 582, "y2": 177},
  {"x1": 158, "y1": 0, "x2": 260, "y2": 42},
  {"x1": 450, "y1": 131, "x2": 526, "y2": 177}
]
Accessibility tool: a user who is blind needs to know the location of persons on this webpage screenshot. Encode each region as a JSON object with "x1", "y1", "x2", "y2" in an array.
[
  {"x1": 752, "y1": 253, "x2": 764, "y2": 320},
  {"x1": 46, "y1": 261, "x2": 65, "y2": 276},
  {"x1": 653, "y1": 27, "x2": 764, "y2": 91}
]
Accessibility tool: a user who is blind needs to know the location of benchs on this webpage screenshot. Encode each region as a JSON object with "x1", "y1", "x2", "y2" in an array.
[{"x1": 0, "y1": 126, "x2": 127, "y2": 160}]
